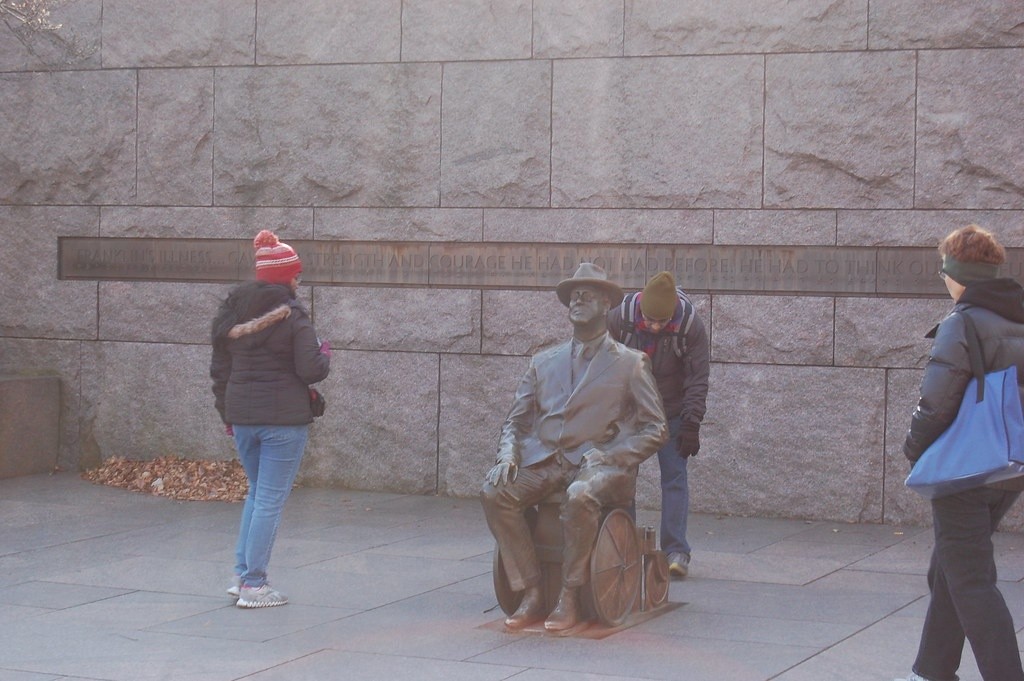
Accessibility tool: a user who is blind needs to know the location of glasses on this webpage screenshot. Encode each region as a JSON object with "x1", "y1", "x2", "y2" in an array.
[
  {"x1": 641, "y1": 313, "x2": 671, "y2": 323},
  {"x1": 938, "y1": 269, "x2": 945, "y2": 279},
  {"x1": 293, "y1": 275, "x2": 303, "y2": 282}
]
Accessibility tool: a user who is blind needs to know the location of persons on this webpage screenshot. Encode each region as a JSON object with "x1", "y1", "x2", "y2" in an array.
[
  {"x1": 208, "y1": 229, "x2": 330, "y2": 607},
  {"x1": 897, "y1": 225, "x2": 1024, "y2": 681},
  {"x1": 606, "y1": 270, "x2": 710, "y2": 576},
  {"x1": 479, "y1": 263, "x2": 671, "y2": 630}
]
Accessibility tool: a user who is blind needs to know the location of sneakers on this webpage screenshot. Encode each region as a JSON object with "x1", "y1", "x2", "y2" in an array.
[
  {"x1": 228, "y1": 574, "x2": 241, "y2": 594},
  {"x1": 236, "y1": 582, "x2": 289, "y2": 607}
]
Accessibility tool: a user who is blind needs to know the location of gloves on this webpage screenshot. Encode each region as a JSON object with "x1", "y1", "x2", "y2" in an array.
[
  {"x1": 319, "y1": 341, "x2": 331, "y2": 358},
  {"x1": 674, "y1": 422, "x2": 700, "y2": 457},
  {"x1": 226, "y1": 423, "x2": 233, "y2": 435}
]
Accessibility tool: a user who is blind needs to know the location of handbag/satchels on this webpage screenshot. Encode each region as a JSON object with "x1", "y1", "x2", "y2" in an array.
[
  {"x1": 905, "y1": 310, "x2": 1024, "y2": 498},
  {"x1": 310, "y1": 387, "x2": 325, "y2": 416}
]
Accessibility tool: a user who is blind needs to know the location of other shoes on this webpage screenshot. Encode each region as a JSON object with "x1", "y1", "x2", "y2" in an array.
[{"x1": 666, "y1": 552, "x2": 689, "y2": 575}]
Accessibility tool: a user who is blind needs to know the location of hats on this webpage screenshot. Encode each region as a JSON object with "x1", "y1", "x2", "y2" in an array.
[
  {"x1": 254, "y1": 229, "x2": 304, "y2": 282},
  {"x1": 640, "y1": 270, "x2": 677, "y2": 319}
]
